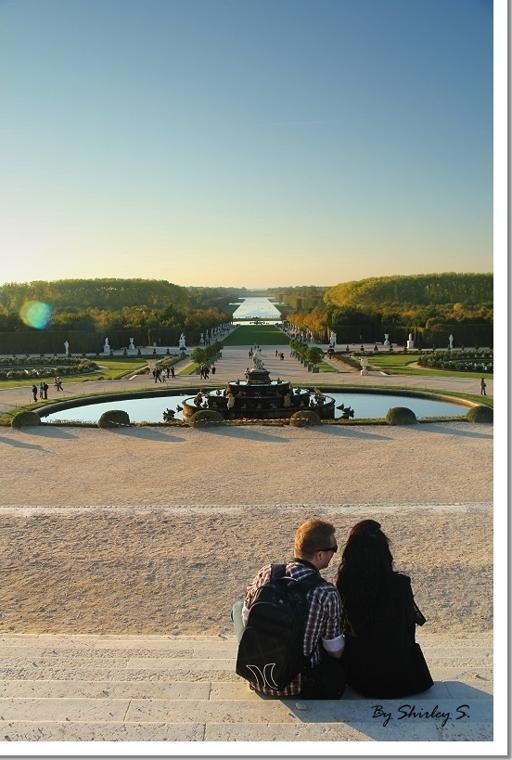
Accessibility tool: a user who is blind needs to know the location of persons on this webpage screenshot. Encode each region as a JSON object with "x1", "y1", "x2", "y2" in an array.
[
  {"x1": 230, "y1": 519, "x2": 434, "y2": 700},
  {"x1": 480, "y1": 378, "x2": 486, "y2": 395},
  {"x1": 153, "y1": 366, "x2": 175, "y2": 383},
  {"x1": 54, "y1": 377, "x2": 63, "y2": 391},
  {"x1": 200, "y1": 365, "x2": 210, "y2": 380},
  {"x1": 275, "y1": 350, "x2": 284, "y2": 359},
  {"x1": 249, "y1": 344, "x2": 261, "y2": 357},
  {"x1": 212, "y1": 366, "x2": 215, "y2": 374},
  {"x1": 32, "y1": 382, "x2": 48, "y2": 402}
]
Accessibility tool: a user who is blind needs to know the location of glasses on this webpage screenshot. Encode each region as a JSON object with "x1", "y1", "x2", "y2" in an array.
[{"x1": 316, "y1": 545, "x2": 337, "y2": 553}]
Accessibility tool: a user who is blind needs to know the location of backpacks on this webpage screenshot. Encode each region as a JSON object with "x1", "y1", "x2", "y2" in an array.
[{"x1": 236, "y1": 563, "x2": 326, "y2": 692}]
